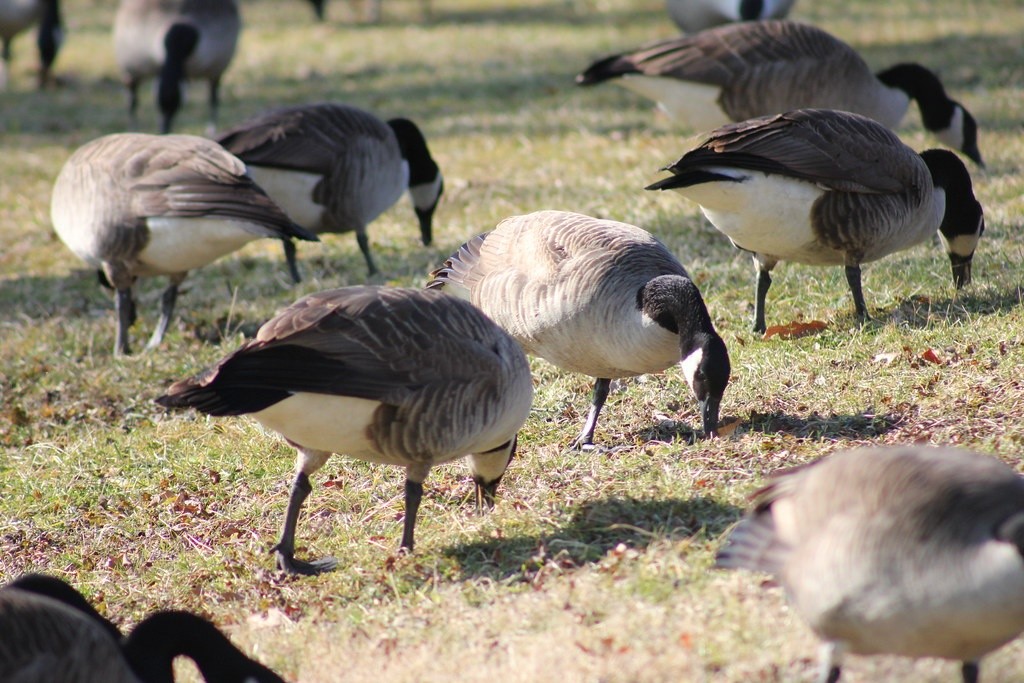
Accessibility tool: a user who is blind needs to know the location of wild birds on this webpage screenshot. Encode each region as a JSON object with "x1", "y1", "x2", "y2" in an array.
[
  {"x1": 154, "y1": 280, "x2": 536, "y2": 573},
  {"x1": 571, "y1": 0, "x2": 989, "y2": 175},
  {"x1": 209, "y1": 102, "x2": 444, "y2": 284},
  {"x1": 431, "y1": 210, "x2": 733, "y2": 451},
  {"x1": 48, "y1": 132, "x2": 320, "y2": 366},
  {"x1": 112, "y1": 1, "x2": 244, "y2": 134},
  {"x1": 712, "y1": 443, "x2": 1024, "y2": 682},
  {"x1": 642, "y1": 106, "x2": 987, "y2": 339},
  {"x1": 0, "y1": 0, "x2": 66, "y2": 95},
  {"x1": 0, "y1": 570, "x2": 289, "y2": 683}
]
